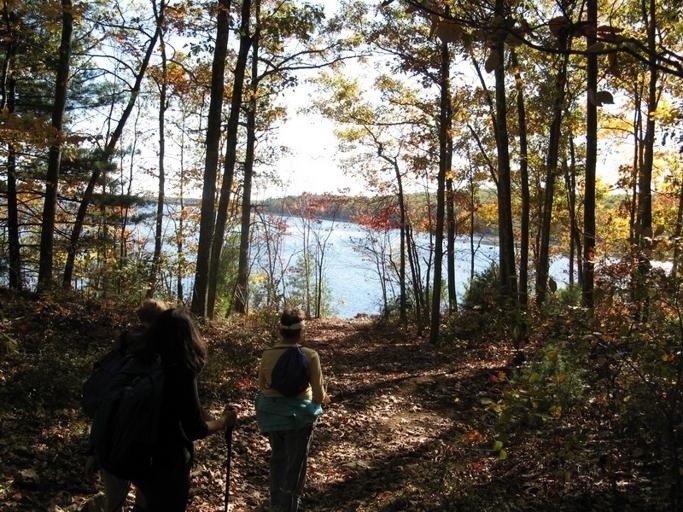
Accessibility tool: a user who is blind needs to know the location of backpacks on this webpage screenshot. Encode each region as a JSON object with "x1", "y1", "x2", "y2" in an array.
[
  {"x1": 270, "y1": 346, "x2": 310, "y2": 395},
  {"x1": 82, "y1": 357, "x2": 163, "y2": 478}
]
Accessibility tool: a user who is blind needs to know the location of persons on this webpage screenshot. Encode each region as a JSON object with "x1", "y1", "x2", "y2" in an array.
[
  {"x1": 84, "y1": 299, "x2": 168, "y2": 512},
  {"x1": 132, "y1": 310, "x2": 237, "y2": 512},
  {"x1": 253, "y1": 308, "x2": 331, "y2": 512}
]
